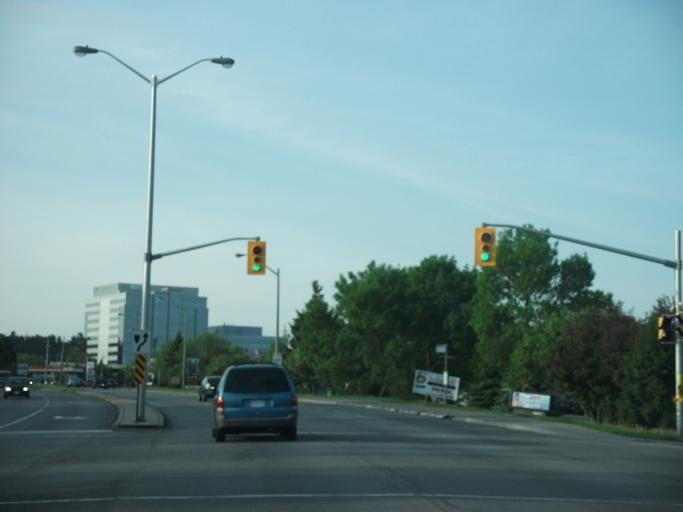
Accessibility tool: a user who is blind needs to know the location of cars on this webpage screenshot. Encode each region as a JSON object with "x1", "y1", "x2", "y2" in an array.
[
  {"x1": 68, "y1": 377, "x2": 81, "y2": 387},
  {"x1": 198, "y1": 364, "x2": 298, "y2": 443},
  {"x1": 3, "y1": 376, "x2": 30, "y2": 399}
]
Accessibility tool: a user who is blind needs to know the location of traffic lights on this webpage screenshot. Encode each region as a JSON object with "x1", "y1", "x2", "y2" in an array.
[
  {"x1": 247, "y1": 241, "x2": 266, "y2": 275},
  {"x1": 474, "y1": 227, "x2": 496, "y2": 267}
]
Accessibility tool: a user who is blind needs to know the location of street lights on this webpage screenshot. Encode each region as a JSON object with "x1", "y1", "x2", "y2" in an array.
[
  {"x1": 73, "y1": 44, "x2": 235, "y2": 421},
  {"x1": 236, "y1": 253, "x2": 282, "y2": 364}
]
